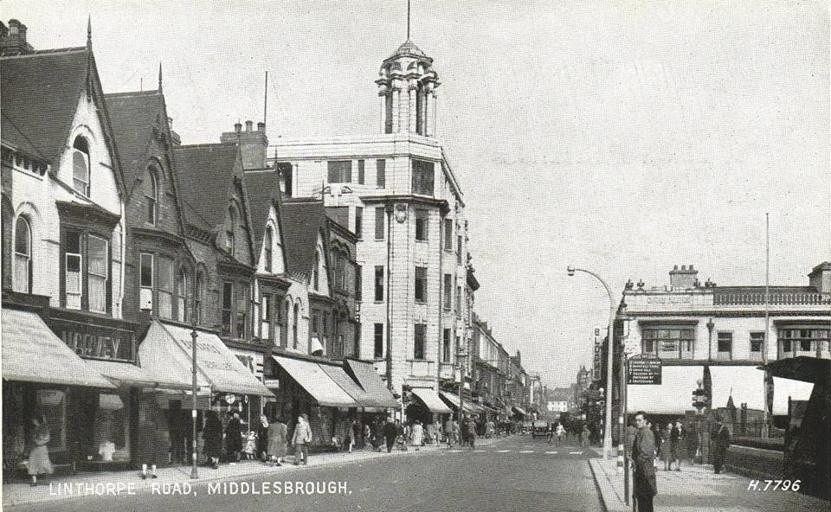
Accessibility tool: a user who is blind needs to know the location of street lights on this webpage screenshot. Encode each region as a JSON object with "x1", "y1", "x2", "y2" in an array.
[
  {"x1": 564, "y1": 264, "x2": 614, "y2": 461},
  {"x1": 167, "y1": 256, "x2": 220, "y2": 478}
]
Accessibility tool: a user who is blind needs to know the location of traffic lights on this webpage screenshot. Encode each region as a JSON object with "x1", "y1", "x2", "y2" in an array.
[
  {"x1": 700, "y1": 390, "x2": 707, "y2": 409},
  {"x1": 691, "y1": 390, "x2": 699, "y2": 410}
]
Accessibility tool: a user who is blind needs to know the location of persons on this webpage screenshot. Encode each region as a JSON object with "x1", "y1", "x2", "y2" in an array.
[
  {"x1": 138, "y1": 416, "x2": 160, "y2": 480},
  {"x1": 626, "y1": 418, "x2": 730, "y2": 474},
  {"x1": 545, "y1": 420, "x2": 568, "y2": 440},
  {"x1": 629, "y1": 411, "x2": 658, "y2": 512},
  {"x1": 203, "y1": 410, "x2": 313, "y2": 469},
  {"x1": 29, "y1": 413, "x2": 53, "y2": 486},
  {"x1": 347, "y1": 411, "x2": 499, "y2": 454}
]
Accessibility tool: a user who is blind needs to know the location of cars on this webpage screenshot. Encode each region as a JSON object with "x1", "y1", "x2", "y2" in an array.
[{"x1": 521, "y1": 419, "x2": 555, "y2": 439}]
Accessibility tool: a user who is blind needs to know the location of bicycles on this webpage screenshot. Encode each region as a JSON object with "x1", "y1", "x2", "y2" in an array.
[
  {"x1": 556, "y1": 436, "x2": 564, "y2": 448},
  {"x1": 546, "y1": 431, "x2": 553, "y2": 446},
  {"x1": 445, "y1": 433, "x2": 455, "y2": 449}
]
